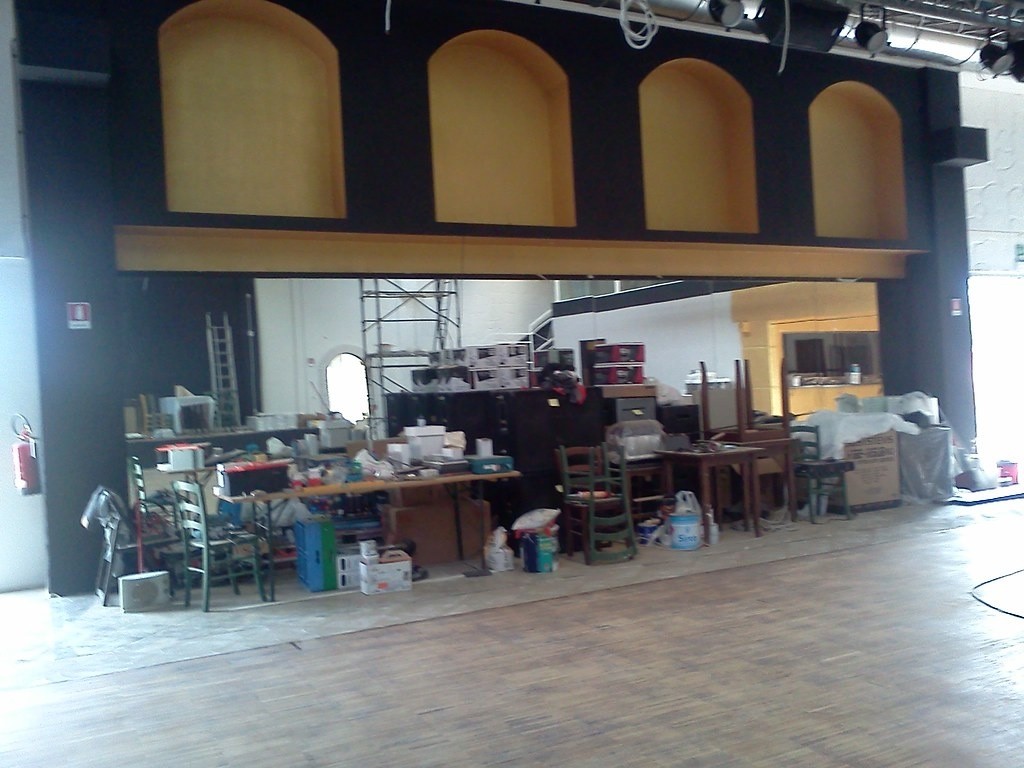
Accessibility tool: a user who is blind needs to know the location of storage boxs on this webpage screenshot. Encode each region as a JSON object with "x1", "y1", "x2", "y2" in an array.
[
  {"x1": 221, "y1": 466, "x2": 287, "y2": 495},
  {"x1": 816, "y1": 411, "x2": 902, "y2": 510},
  {"x1": 294, "y1": 518, "x2": 337, "y2": 594},
  {"x1": 386, "y1": 481, "x2": 471, "y2": 508},
  {"x1": 593, "y1": 365, "x2": 644, "y2": 385},
  {"x1": 592, "y1": 342, "x2": 645, "y2": 365},
  {"x1": 153, "y1": 443, "x2": 204, "y2": 473},
  {"x1": 344, "y1": 437, "x2": 408, "y2": 464},
  {"x1": 594, "y1": 384, "x2": 657, "y2": 398},
  {"x1": 382, "y1": 499, "x2": 491, "y2": 565},
  {"x1": 358, "y1": 555, "x2": 413, "y2": 596},
  {"x1": 215, "y1": 462, "x2": 253, "y2": 487},
  {"x1": 337, "y1": 543, "x2": 361, "y2": 590},
  {"x1": 410, "y1": 342, "x2": 575, "y2": 389},
  {"x1": 404, "y1": 425, "x2": 446, "y2": 459},
  {"x1": 612, "y1": 397, "x2": 657, "y2": 426}
]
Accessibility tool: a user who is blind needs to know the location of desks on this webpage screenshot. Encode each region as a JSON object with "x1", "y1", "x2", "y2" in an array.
[
  {"x1": 151, "y1": 453, "x2": 521, "y2": 602},
  {"x1": 654, "y1": 358, "x2": 801, "y2": 539}
]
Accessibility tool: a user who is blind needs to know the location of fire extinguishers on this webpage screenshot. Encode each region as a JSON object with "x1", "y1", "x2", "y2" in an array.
[{"x1": 10, "y1": 414, "x2": 41, "y2": 497}]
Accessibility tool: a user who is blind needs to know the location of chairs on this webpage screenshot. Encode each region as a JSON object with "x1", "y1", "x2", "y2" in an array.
[
  {"x1": 552, "y1": 440, "x2": 675, "y2": 564},
  {"x1": 169, "y1": 479, "x2": 268, "y2": 612},
  {"x1": 786, "y1": 421, "x2": 855, "y2": 524},
  {"x1": 92, "y1": 488, "x2": 181, "y2": 608}
]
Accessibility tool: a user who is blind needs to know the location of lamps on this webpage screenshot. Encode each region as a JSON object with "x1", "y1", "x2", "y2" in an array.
[
  {"x1": 855, "y1": 2, "x2": 891, "y2": 55},
  {"x1": 979, "y1": 23, "x2": 1015, "y2": 77},
  {"x1": 708, "y1": 0, "x2": 746, "y2": 28}
]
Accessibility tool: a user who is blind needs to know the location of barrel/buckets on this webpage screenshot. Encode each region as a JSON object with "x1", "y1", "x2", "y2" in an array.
[
  {"x1": 521, "y1": 523, "x2": 560, "y2": 573},
  {"x1": 1002, "y1": 463, "x2": 1018, "y2": 484},
  {"x1": 669, "y1": 513, "x2": 702, "y2": 550}
]
